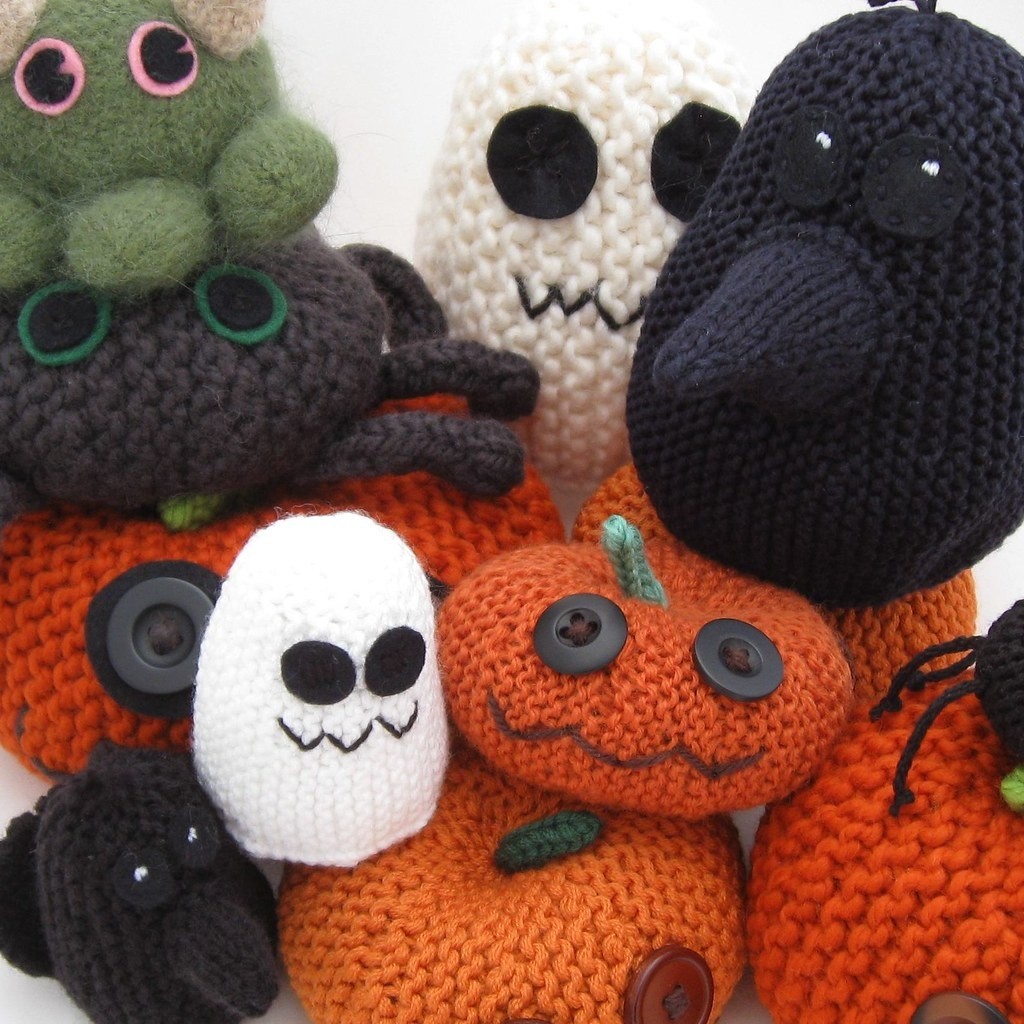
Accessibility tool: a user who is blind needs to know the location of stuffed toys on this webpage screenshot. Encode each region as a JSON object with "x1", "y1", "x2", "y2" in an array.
[{"x1": 0, "y1": 0, "x2": 1023, "y2": 1022}]
